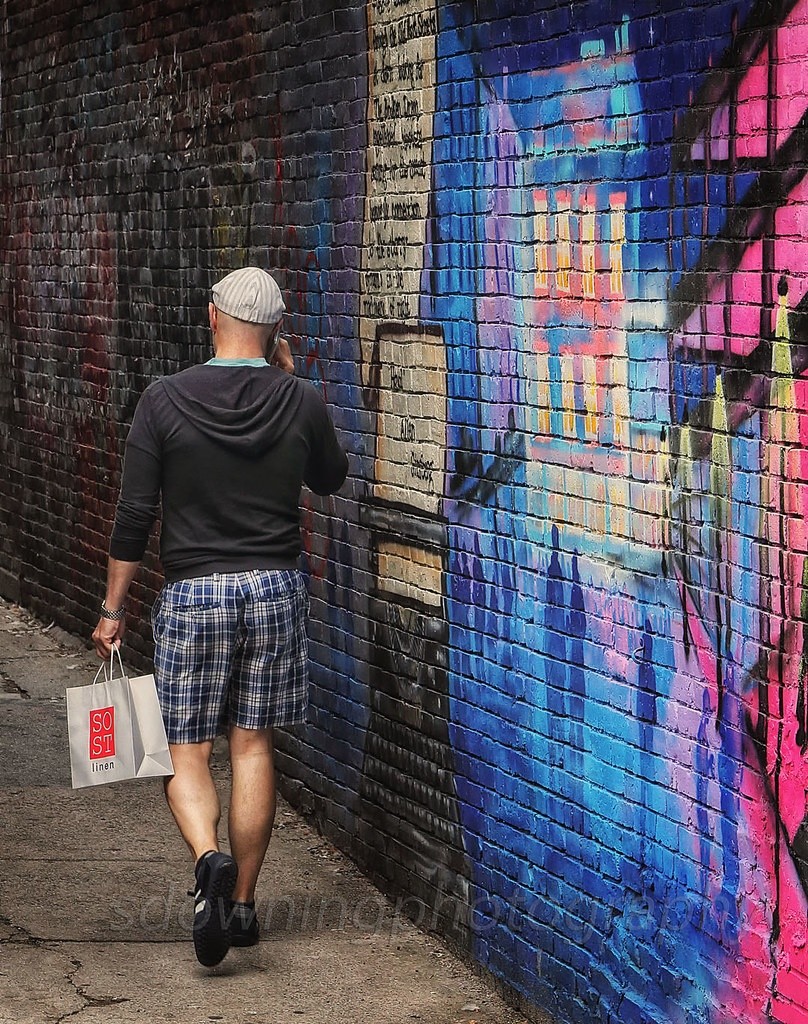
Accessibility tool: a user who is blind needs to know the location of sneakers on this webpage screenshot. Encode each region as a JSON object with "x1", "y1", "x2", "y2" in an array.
[
  {"x1": 187, "y1": 851, "x2": 238, "y2": 967},
  {"x1": 231, "y1": 900, "x2": 258, "y2": 948}
]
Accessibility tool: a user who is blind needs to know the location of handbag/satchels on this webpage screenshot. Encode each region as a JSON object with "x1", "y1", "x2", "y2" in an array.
[{"x1": 66, "y1": 641, "x2": 175, "y2": 790}]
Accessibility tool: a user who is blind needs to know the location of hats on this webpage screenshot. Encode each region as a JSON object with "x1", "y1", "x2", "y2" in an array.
[{"x1": 212, "y1": 267, "x2": 285, "y2": 323}]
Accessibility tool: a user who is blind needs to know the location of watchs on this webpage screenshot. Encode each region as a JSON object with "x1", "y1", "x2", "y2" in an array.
[{"x1": 99, "y1": 600, "x2": 124, "y2": 620}]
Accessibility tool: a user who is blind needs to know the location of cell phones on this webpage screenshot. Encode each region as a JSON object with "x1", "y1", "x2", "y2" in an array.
[{"x1": 265, "y1": 328, "x2": 281, "y2": 361}]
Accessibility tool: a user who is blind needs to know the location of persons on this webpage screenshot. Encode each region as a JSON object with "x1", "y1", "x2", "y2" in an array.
[{"x1": 94, "y1": 266, "x2": 351, "y2": 966}]
